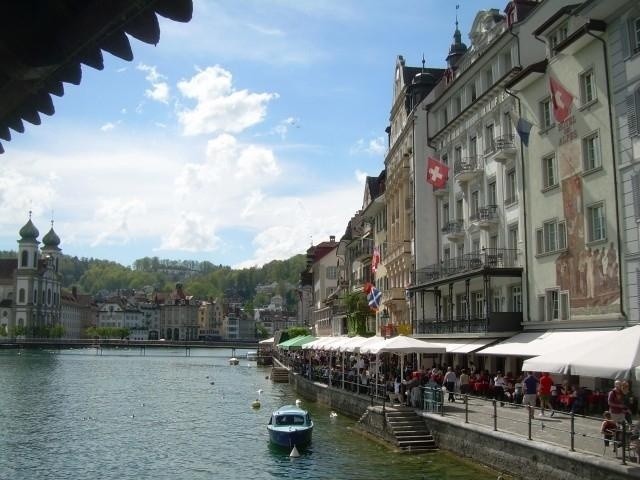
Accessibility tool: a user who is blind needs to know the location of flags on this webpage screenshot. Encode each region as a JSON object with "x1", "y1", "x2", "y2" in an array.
[
  {"x1": 372, "y1": 247, "x2": 380, "y2": 272},
  {"x1": 548, "y1": 73, "x2": 572, "y2": 125},
  {"x1": 364, "y1": 283, "x2": 381, "y2": 310},
  {"x1": 427, "y1": 157, "x2": 448, "y2": 188},
  {"x1": 508, "y1": 110, "x2": 532, "y2": 147}
]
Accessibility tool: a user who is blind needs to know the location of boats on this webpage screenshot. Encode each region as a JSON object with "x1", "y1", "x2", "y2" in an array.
[
  {"x1": 265, "y1": 399, "x2": 315, "y2": 447},
  {"x1": 229, "y1": 357, "x2": 239, "y2": 366},
  {"x1": 246, "y1": 351, "x2": 257, "y2": 361}
]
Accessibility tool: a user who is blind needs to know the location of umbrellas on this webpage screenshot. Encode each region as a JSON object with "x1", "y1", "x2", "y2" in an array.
[{"x1": 276, "y1": 335, "x2": 445, "y2": 401}]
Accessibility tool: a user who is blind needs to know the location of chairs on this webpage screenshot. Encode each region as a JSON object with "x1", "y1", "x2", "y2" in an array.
[
  {"x1": 602, "y1": 422, "x2": 639, "y2": 464},
  {"x1": 309, "y1": 361, "x2": 639, "y2": 413}
]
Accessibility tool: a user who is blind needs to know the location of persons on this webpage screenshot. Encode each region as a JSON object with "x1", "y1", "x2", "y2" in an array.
[
  {"x1": 522, "y1": 372, "x2": 586, "y2": 419},
  {"x1": 279, "y1": 349, "x2": 444, "y2": 406},
  {"x1": 555, "y1": 241, "x2": 620, "y2": 299},
  {"x1": 404, "y1": 360, "x2": 523, "y2": 403},
  {"x1": 601, "y1": 381, "x2": 633, "y2": 451}
]
